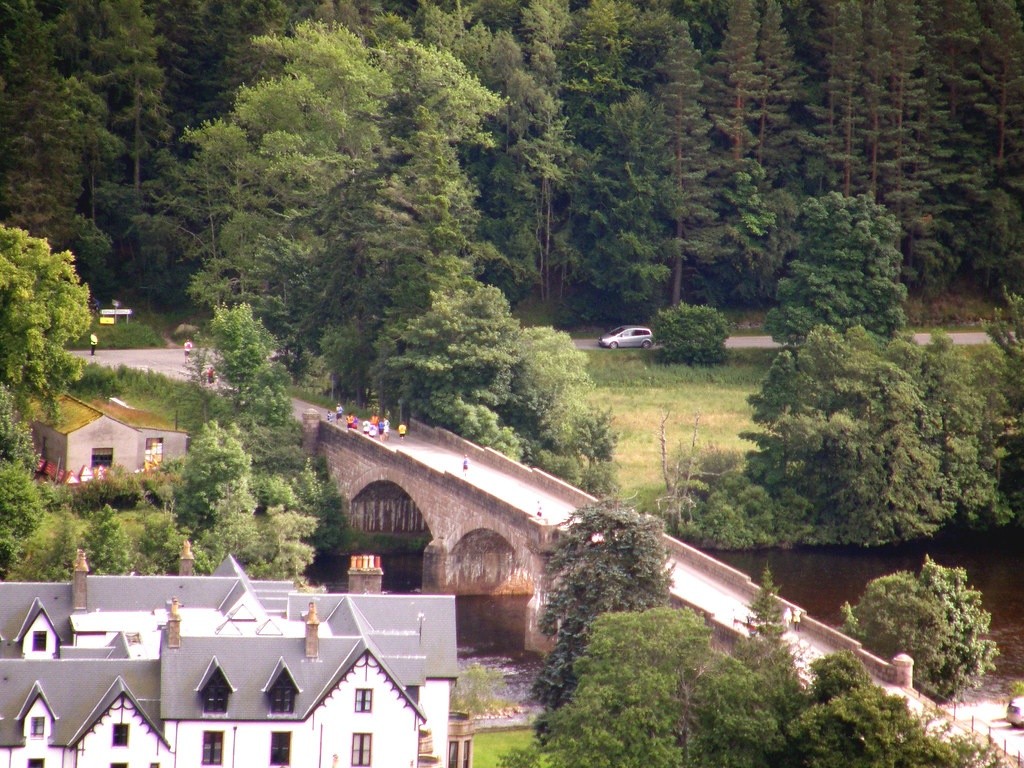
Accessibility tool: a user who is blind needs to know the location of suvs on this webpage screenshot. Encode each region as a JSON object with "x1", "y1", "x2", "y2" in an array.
[{"x1": 598, "y1": 324, "x2": 654, "y2": 348}]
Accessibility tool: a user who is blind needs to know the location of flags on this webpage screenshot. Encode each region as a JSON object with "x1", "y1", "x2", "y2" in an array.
[{"x1": 112, "y1": 300, "x2": 121, "y2": 306}]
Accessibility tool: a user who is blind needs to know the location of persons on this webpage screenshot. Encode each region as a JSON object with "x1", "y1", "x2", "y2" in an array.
[
  {"x1": 742, "y1": 606, "x2": 803, "y2": 638},
  {"x1": 536, "y1": 500, "x2": 543, "y2": 519},
  {"x1": 463, "y1": 453, "x2": 469, "y2": 478},
  {"x1": 327, "y1": 403, "x2": 390, "y2": 442},
  {"x1": 398, "y1": 420, "x2": 407, "y2": 442},
  {"x1": 90, "y1": 297, "x2": 100, "y2": 316},
  {"x1": 90, "y1": 330, "x2": 98, "y2": 357},
  {"x1": 184, "y1": 339, "x2": 214, "y2": 388}
]
